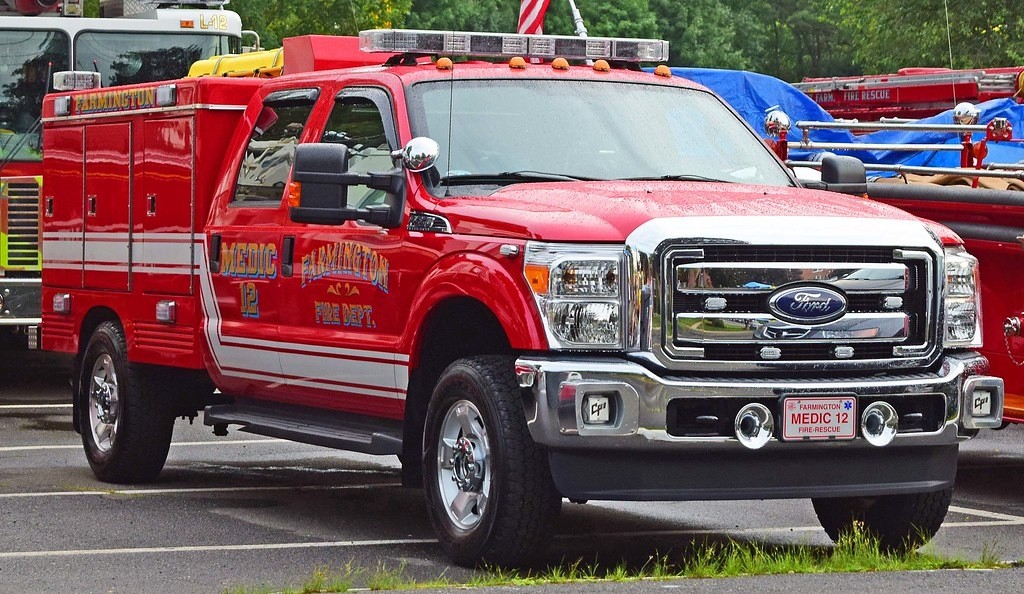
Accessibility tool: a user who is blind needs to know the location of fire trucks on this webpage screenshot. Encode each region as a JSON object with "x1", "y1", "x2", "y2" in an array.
[
  {"x1": 0, "y1": 0, "x2": 256, "y2": 352},
  {"x1": 41, "y1": 29, "x2": 1006, "y2": 572}
]
